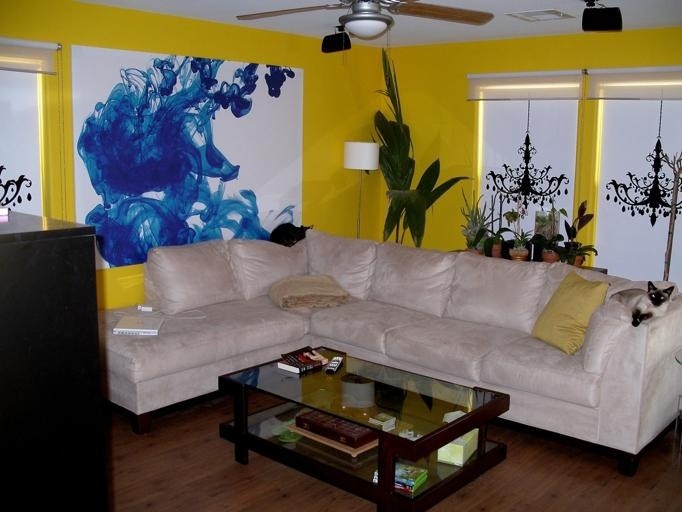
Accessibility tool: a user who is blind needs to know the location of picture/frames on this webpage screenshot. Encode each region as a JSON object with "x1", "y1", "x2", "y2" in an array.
[{"x1": 69, "y1": 43, "x2": 304, "y2": 271}]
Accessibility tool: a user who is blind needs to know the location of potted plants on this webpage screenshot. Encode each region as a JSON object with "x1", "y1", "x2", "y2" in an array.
[
  {"x1": 508, "y1": 230, "x2": 533, "y2": 261},
  {"x1": 530, "y1": 233, "x2": 564, "y2": 262},
  {"x1": 473, "y1": 227, "x2": 513, "y2": 258},
  {"x1": 564, "y1": 200, "x2": 598, "y2": 267},
  {"x1": 460, "y1": 188, "x2": 500, "y2": 256}
]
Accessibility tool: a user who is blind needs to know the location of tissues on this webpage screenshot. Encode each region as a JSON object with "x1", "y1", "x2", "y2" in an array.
[{"x1": 437, "y1": 411, "x2": 479, "y2": 467}]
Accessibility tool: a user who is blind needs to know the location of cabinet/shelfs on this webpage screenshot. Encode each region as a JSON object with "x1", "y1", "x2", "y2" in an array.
[{"x1": 0, "y1": 212, "x2": 113, "y2": 512}]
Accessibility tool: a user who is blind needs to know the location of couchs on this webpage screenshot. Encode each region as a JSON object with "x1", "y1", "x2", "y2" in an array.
[
  {"x1": 97, "y1": 237, "x2": 377, "y2": 435},
  {"x1": 309, "y1": 241, "x2": 682, "y2": 478}
]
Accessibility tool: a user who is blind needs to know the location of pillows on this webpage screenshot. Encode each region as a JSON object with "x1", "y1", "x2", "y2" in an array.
[
  {"x1": 530, "y1": 271, "x2": 610, "y2": 357},
  {"x1": 228, "y1": 239, "x2": 307, "y2": 301},
  {"x1": 604, "y1": 279, "x2": 679, "y2": 307},
  {"x1": 581, "y1": 298, "x2": 632, "y2": 373},
  {"x1": 444, "y1": 250, "x2": 547, "y2": 337},
  {"x1": 539, "y1": 261, "x2": 633, "y2": 303},
  {"x1": 148, "y1": 240, "x2": 241, "y2": 316},
  {"x1": 306, "y1": 228, "x2": 375, "y2": 302},
  {"x1": 369, "y1": 242, "x2": 455, "y2": 320}
]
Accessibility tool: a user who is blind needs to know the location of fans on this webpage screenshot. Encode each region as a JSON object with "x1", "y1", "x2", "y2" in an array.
[{"x1": 235, "y1": 0, "x2": 494, "y2": 41}]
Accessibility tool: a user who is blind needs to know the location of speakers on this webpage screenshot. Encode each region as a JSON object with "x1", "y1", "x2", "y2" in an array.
[
  {"x1": 581, "y1": 7, "x2": 623, "y2": 33},
  {"x1": 321, "y1": 31, "x2": 352, "y2": 52}
]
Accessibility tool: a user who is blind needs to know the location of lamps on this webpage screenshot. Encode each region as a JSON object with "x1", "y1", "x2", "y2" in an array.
[{"x1": 343, "y1": 141, "x2": 380, "y2": 172}]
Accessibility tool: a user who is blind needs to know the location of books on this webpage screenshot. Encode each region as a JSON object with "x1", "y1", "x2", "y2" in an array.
[
  {"x1": 372, "y1": 462, "x2": 428, "y2": 492},
  {"x1": 112, "y1": 314, "x2": 165, "y2": 337},
  {"x1": 277, "y1": 346, "x2": 328, "y2": 374}
]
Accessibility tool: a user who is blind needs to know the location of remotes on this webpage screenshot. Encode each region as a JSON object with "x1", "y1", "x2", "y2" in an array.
[{"x1": 325, "y1": 356, "x2": 343, "y2": 373}]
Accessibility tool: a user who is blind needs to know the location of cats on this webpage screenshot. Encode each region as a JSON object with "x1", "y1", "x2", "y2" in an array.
[{"x1": 609, "y1": 280, "x2": 676, "y2": 328}]
